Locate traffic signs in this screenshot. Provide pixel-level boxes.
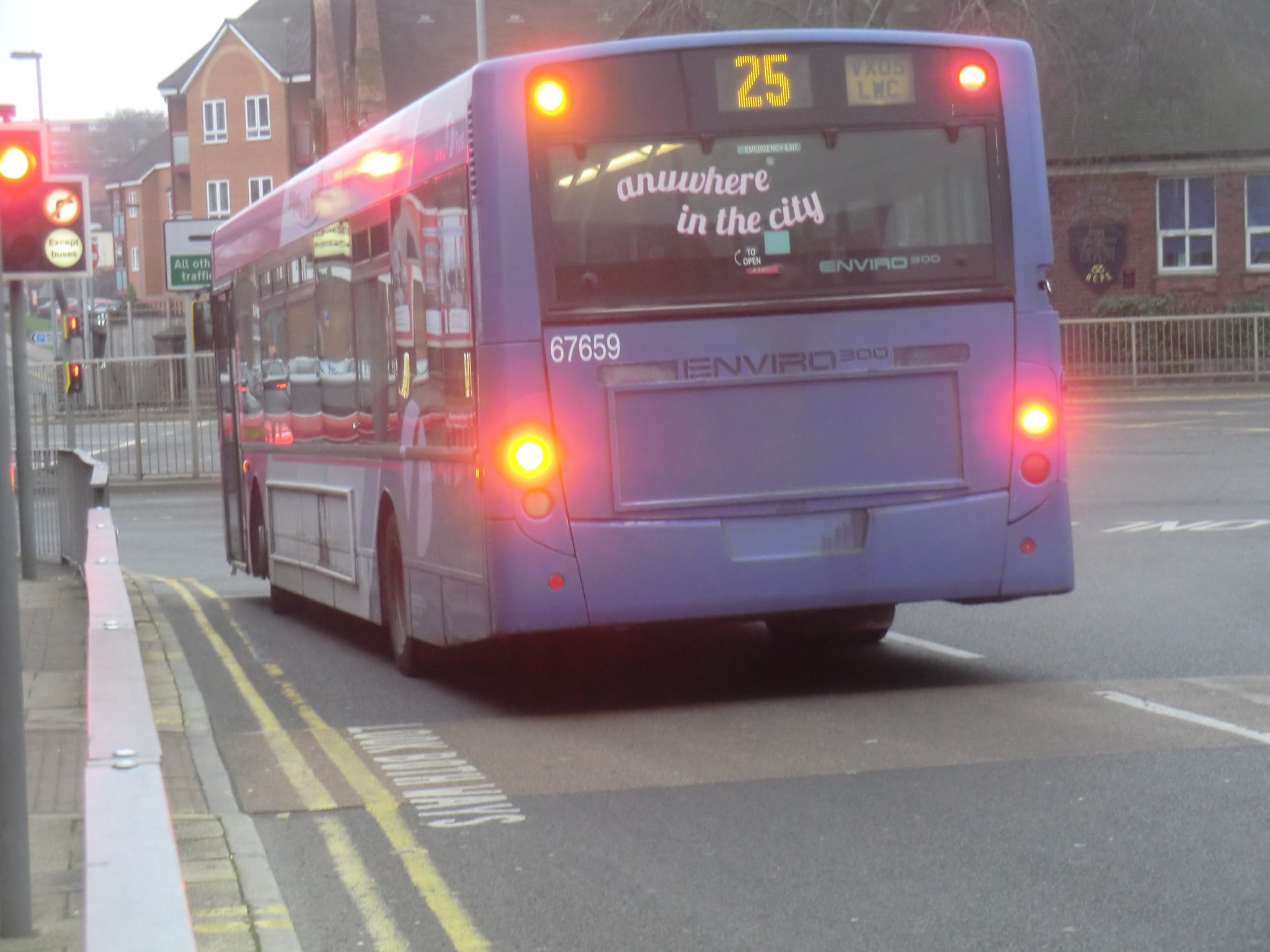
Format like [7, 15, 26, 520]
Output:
[163, 217, 229, 291]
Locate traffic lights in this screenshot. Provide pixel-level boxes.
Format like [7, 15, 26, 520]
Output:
[0, 119, 50, 281]
[70, 364, 83, 391]
[69, 316, 81, 337]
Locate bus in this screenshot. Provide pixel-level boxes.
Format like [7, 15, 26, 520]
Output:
[189, 26, 1075, 676]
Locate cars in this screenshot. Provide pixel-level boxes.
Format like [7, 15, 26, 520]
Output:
[36, 296, 157, 325]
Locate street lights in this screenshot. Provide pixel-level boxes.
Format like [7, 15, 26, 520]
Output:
[11, 51, 45, 119]
